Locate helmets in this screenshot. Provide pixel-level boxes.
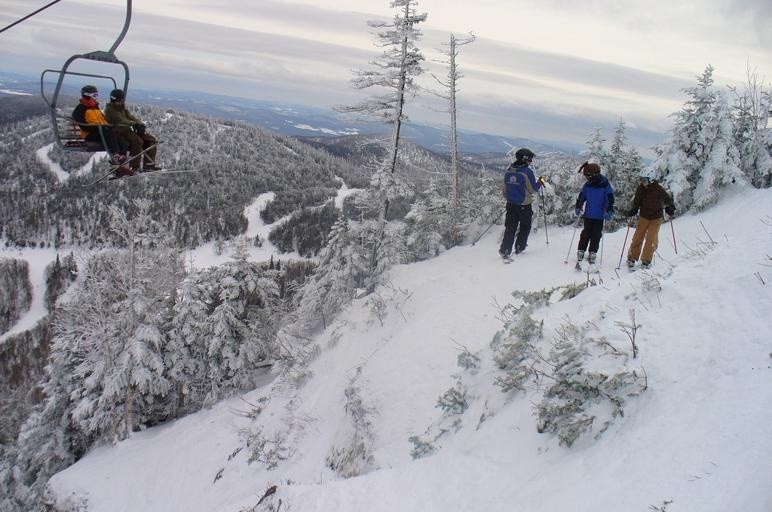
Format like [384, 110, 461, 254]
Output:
[583, 164, 600, 177]
[516, 149, 534, 161]
[640, 167, 654, 183]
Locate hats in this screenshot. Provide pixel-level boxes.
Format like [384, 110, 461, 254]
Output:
[111, 89, 125, 100]
[81, 86, 97, 98]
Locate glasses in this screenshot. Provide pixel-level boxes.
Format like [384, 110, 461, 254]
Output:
[85, 93, 98, 99]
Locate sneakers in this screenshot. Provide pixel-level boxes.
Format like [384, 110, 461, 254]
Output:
[578, 251, 596, 259]
[144, 165, 161, 171]
[627, 260, 649, 267]
[501, 248, 522, 258]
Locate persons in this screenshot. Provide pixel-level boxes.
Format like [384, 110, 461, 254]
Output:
[497, 147, 547, 258]
[71, 84, 134, 177]
[102, 88, 163, 173]
[574, 163, 616, 264]
[626, 165, 678, 268]
